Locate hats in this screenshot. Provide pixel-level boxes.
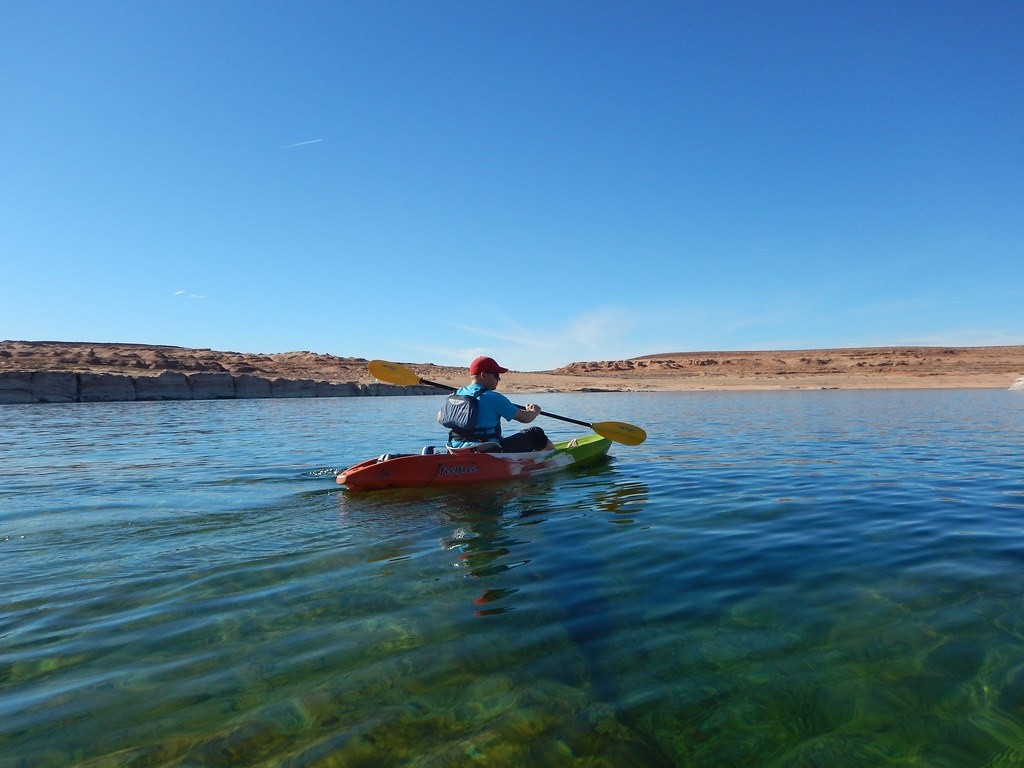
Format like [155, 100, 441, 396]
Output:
[470, 356, 508, 375]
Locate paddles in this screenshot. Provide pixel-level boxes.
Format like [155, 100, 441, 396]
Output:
[368, 360, 647, 446]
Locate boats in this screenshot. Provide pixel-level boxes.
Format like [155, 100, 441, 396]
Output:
[336, 434, 612, 493]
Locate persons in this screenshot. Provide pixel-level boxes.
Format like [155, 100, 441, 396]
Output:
[447, 356, 578, 453]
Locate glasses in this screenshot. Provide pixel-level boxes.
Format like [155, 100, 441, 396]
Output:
[485, 372, 499, 379]
